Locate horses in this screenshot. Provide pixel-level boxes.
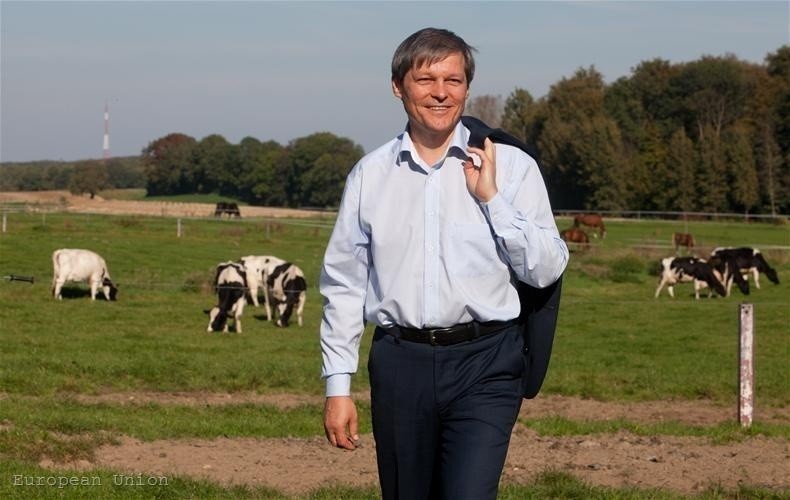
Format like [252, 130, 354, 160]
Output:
[214, 202, 241, 219]
[671, 232, 697, 255]
[560, 213, 607, 254]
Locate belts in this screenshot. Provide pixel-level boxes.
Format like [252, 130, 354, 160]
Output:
[395, 324, 479, 345]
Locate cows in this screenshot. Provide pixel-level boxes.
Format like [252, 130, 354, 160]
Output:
[50, 248, 118, 301]
[202, 254, 308, 335]
[653, 247, 779, 300]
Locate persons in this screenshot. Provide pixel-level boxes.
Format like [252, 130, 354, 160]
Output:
[321, 27, 568, 500]
